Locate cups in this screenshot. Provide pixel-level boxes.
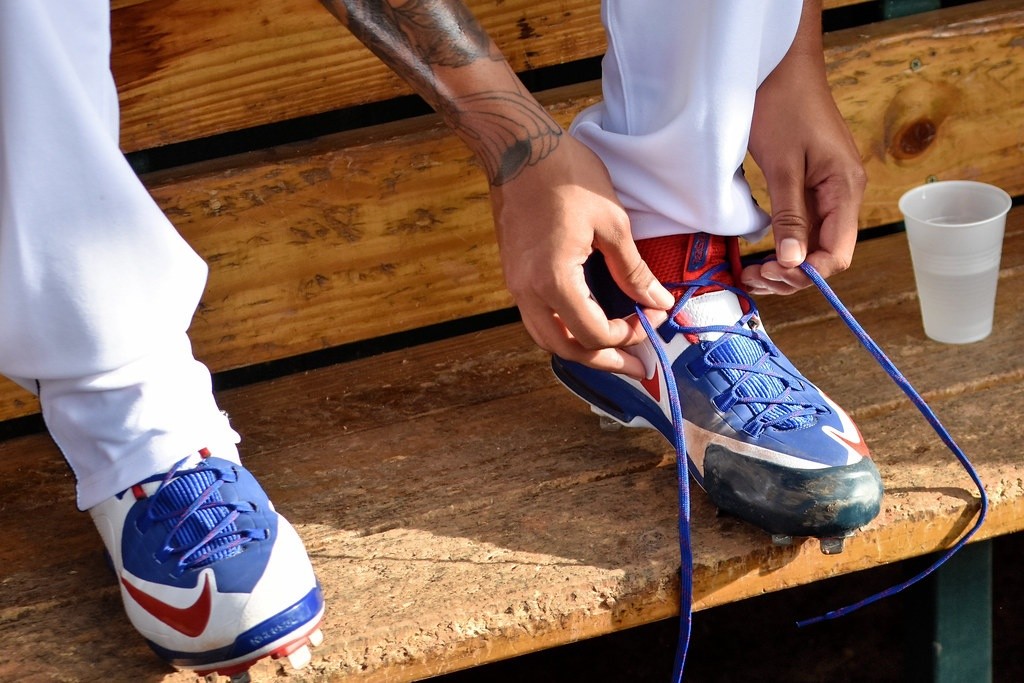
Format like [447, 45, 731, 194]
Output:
[898, 179, 1013, 343]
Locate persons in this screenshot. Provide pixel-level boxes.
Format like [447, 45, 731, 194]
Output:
[0, 0, 885, 678]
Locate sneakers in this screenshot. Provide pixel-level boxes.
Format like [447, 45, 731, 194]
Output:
[546, 229, 884, 553]
[86, 439, 326, 680]
